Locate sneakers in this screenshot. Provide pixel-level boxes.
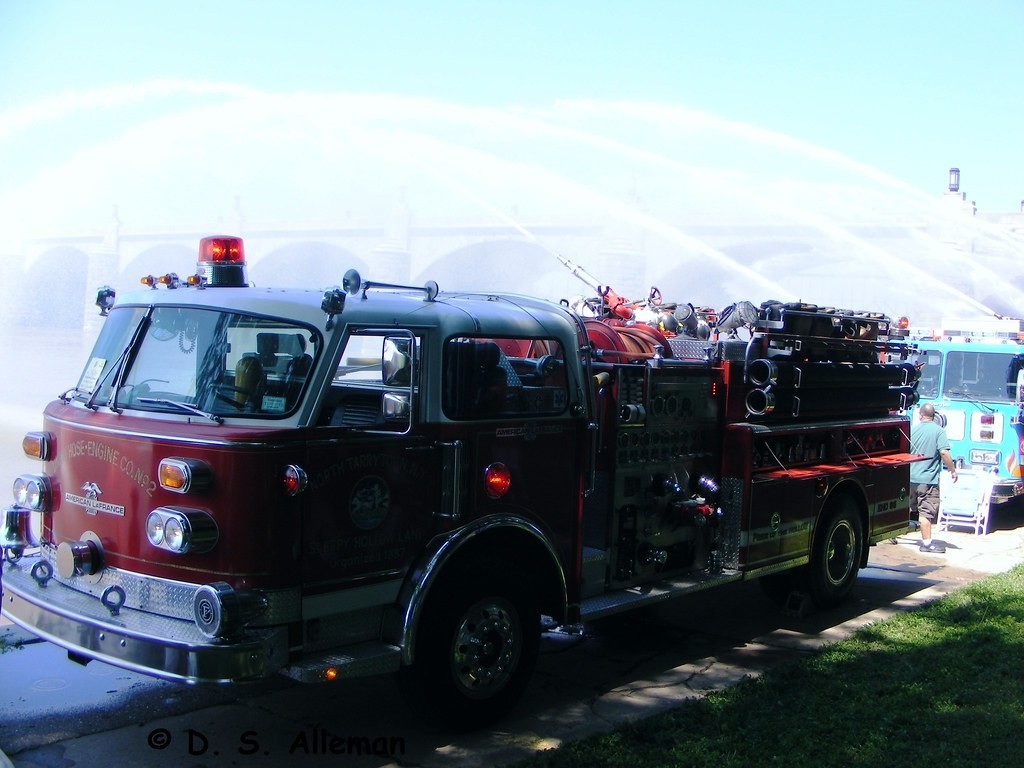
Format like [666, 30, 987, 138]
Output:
[889, 534, 898, 544]
[920, 540, 946, 553]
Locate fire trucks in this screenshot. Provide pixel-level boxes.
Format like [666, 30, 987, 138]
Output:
[876, 311, 1024, 537]
[0, 232, 934, 738]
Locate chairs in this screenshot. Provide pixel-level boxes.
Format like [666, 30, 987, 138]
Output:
[939, 468, 994, 537]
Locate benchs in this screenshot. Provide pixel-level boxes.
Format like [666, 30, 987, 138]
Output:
[205, 374, 411, 435]
